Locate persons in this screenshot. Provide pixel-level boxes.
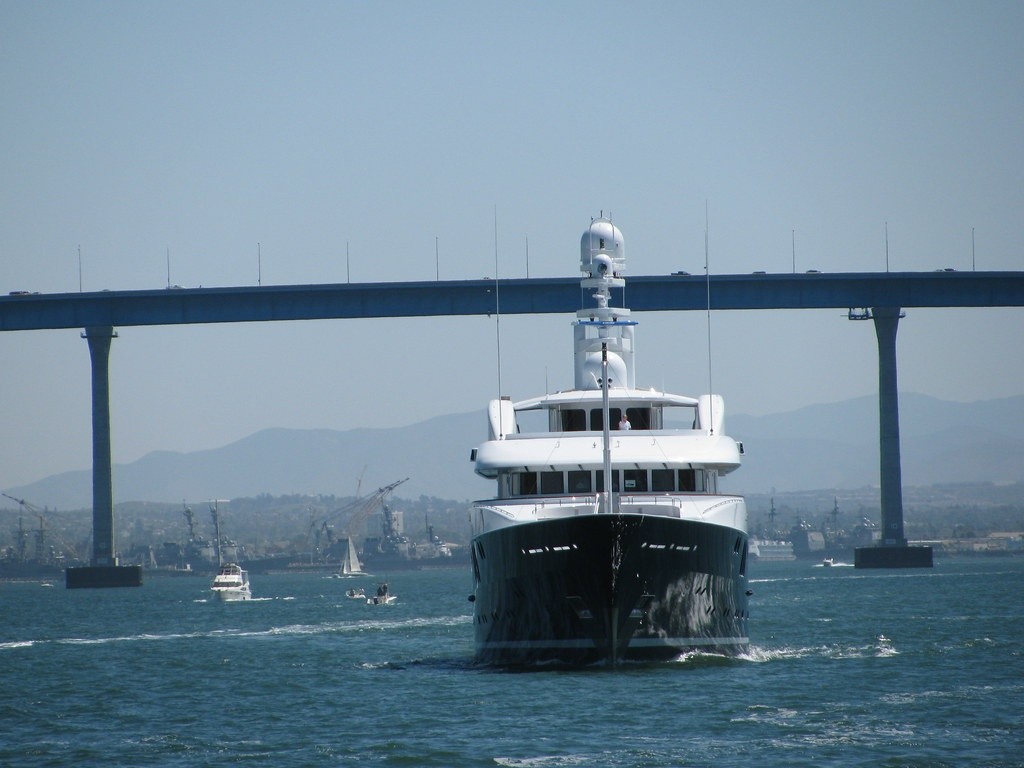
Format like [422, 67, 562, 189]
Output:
[618, 414, 632, 430]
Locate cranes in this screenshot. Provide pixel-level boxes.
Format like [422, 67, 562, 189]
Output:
[0, 494, 85, 557]
[305, 474, 416, 552]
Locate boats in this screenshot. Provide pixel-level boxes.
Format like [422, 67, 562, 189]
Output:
[823, 558, 833, 567]
[364, 580, 397, 605]
[345, 589, 365, 600]
[207, 563, 253, 604]
[464, 204, 748, 667]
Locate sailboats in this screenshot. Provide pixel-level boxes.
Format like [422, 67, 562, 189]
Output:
[332, 539, 368, 578]
